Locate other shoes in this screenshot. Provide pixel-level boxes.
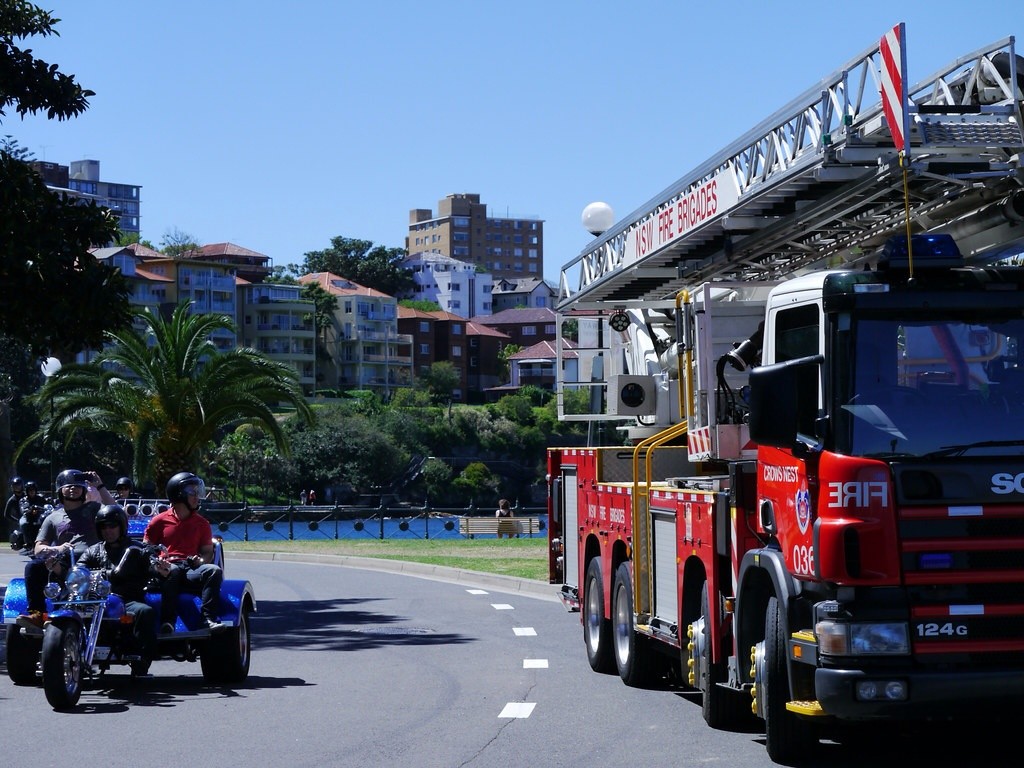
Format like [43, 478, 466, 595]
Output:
[206, 619, 227, 634]
[160, 623, 174, 637]
[19, 547, 34, 556]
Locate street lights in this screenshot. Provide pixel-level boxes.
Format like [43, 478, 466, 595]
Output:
[581, 201, 614, 446]
[41, 357, 62, 504]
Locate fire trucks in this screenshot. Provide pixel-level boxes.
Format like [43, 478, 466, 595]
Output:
[543, 22, 1024, 764]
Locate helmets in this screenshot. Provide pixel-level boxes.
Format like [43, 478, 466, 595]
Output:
[166, 471, 206, 503]
[10, 475, 25, 486]
[25, 481, 37, 493]
[115, 476, 134, 490]
[95, 504, 128, 541]
[55, 469, 88, 493]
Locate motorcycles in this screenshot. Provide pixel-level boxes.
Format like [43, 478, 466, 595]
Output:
[1, 538, 263, 709]
[117, 498, 173, 542]
[9, 504, 61, 559]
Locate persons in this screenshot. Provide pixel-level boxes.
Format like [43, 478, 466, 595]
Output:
[143, 471, 227, 634]
[114, 477, 143, 499]
[19, 481, 47, 550]
[16, 469, 129, 634]
[4, 476, 27, 524]
[310, 490, 316, 505]
[300, 490, 307, 506]
[496, 499, 514, 538]
[45, 505, 171, 681]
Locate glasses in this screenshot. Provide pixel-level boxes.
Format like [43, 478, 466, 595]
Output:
[117, 486, 129, 491]
[185, 490, 200, 496]
[100, 521, 119, 530]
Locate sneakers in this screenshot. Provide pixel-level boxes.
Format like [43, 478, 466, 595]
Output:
[16, 610, 45, 634]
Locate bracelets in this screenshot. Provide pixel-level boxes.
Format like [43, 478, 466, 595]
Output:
[97, 485, 104, 490]
